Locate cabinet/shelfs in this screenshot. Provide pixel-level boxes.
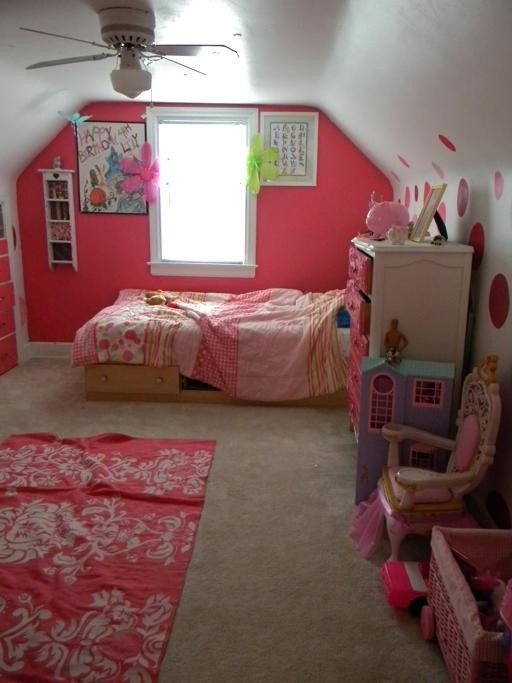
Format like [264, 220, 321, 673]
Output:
[39, 167, 78, 270]
[0, 239, 18, 375]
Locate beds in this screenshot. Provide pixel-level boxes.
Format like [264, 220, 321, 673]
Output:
[67, 287, 350, 408]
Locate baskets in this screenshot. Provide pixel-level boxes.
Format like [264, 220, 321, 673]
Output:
[428, 526, 512, 683]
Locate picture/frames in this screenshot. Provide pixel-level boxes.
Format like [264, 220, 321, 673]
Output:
[77, 119, 149, 215]
[408, 181, 449, 244]
[256, 110, 317, 186]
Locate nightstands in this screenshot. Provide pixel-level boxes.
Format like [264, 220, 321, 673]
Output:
[343, 236, 476, 443]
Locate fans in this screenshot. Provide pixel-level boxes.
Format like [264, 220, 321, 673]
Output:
[18, 1, 243, 100]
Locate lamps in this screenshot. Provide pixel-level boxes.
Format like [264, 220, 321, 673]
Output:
[111, 48, 151, 100]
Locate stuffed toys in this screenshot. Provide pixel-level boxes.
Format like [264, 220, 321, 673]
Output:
[144, 290, 181, 305]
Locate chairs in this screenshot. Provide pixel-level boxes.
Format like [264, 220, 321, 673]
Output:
[348, 354, 502, 562]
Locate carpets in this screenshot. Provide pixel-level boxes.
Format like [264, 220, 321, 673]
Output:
[1, 431, 216, 682]
